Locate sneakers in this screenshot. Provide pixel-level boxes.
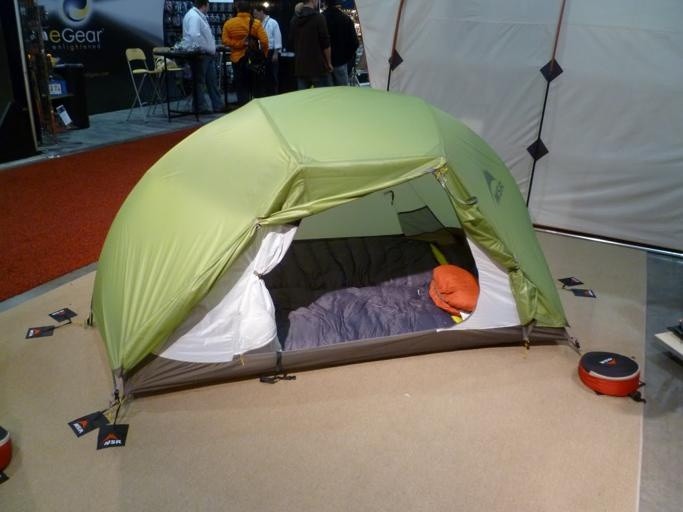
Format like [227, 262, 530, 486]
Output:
[192, 105, 235, 114]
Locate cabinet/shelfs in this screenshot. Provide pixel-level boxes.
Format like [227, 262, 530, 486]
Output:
[46, 63, 90, 133]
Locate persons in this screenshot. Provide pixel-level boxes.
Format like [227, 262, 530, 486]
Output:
[180, 1, 223, 115]
[219, 1, 370, 113]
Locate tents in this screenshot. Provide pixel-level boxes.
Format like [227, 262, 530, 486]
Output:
[86, 85, 587, 406]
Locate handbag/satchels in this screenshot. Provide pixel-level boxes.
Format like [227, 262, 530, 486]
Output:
[248, 49, 266, 69]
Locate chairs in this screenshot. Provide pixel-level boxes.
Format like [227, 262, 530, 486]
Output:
[125, 44, 226, 124]
